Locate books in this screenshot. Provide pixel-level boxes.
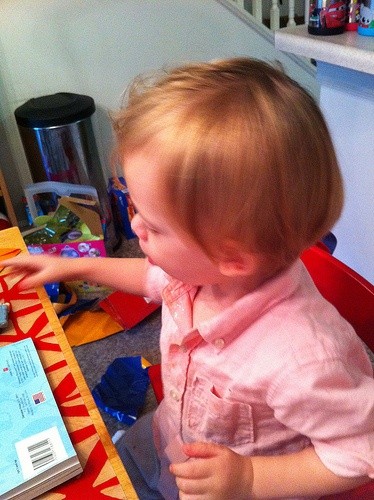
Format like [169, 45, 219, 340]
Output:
[1, 335, 85, 500]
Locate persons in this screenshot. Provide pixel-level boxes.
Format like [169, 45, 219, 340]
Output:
[0, 53, 374, 499]
